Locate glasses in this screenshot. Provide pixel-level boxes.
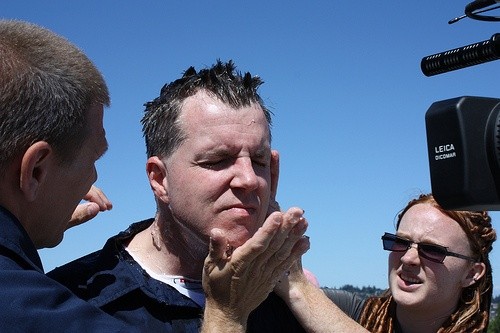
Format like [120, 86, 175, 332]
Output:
[381, 232, 478, 263]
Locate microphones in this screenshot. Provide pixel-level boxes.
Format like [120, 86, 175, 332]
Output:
[421, 33, 500, 77]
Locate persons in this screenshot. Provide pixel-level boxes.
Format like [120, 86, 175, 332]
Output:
[0, 15, 312, 333]
[42, 57, 305, 333]
[265, 150, 498, 333]
